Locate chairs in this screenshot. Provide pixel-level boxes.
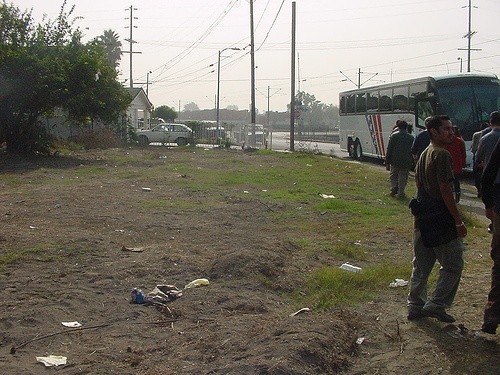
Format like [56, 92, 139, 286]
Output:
[351, 95, 407, 110]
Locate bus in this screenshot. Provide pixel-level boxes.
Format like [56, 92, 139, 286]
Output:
[338, 71, 500, 172]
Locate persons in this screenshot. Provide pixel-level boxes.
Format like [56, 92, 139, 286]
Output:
[470, 111, 500, 335]
[406, 115, 467, 323]
[386, 120, 414, 198]
[410, 117, 432, 163]
[445, 136, 466, 202]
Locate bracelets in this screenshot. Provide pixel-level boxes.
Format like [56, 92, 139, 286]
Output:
[456, 221, 464, 226]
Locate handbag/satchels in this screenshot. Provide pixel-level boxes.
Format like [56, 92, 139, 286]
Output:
[412, 198, 457, 247]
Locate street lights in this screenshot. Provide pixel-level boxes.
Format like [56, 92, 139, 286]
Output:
[217, 47, 240, 145]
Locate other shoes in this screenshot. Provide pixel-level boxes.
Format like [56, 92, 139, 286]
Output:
[399, 194, 404, 198]
[390, 192, 397, 196]
[420, 308, 455, 322]
[481, 321, 498, 334]
[407, 312, 420, 320]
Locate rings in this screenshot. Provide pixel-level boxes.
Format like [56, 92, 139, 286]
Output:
[464, 233, 466, 236]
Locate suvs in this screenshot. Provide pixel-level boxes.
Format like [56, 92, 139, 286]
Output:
[135, 123, 192, 147]
[200, 121, 225, 139]
[240, 124, 266, 145]
